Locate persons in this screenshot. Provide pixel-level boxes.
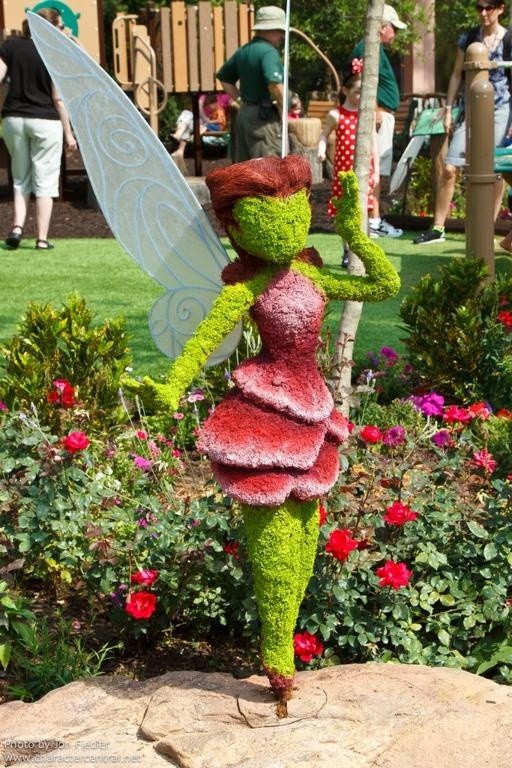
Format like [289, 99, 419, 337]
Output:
[168, 90, 303, 158]
[341, 4, 408, 239]
[316, 61, 376, 269]
[215, 5, 289, 163]
[0, 8, 79, 250]
[412, 0, 512, 246]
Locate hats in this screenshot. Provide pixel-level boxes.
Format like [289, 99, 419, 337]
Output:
[251, 6, 293, 32]
[383, 3, 408, 29]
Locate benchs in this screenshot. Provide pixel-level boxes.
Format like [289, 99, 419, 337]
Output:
[303, 99, 414, 159]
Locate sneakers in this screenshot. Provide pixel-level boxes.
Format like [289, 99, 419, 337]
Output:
[369, 218, 403, 239]
[413, 226, 446, 244]
[342, 250, 349, 267]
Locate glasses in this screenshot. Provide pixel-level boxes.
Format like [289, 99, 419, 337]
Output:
[476, 5, 496, 11]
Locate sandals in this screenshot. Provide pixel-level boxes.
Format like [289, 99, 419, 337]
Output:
[5, 226, 24, 246]
[35, 239, 54, 249]
